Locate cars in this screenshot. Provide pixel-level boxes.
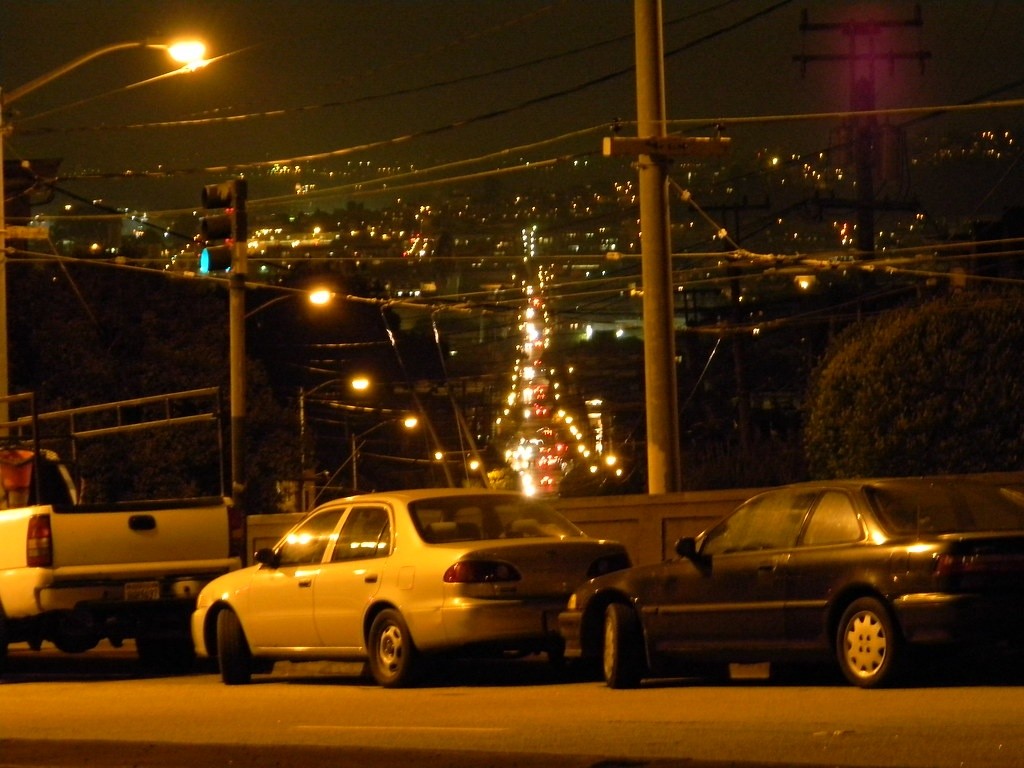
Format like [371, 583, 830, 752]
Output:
[190, 488, 634, 689]
[554, 476, 1024, 692]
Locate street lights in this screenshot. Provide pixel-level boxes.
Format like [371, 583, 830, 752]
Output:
[0, 36, 207, 440]
[294, 373, 370, 514]
[348, 411, 421, 501]
[229, 177, 339, 508]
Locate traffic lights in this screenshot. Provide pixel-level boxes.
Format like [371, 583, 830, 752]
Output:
[199, 182, 233, 277]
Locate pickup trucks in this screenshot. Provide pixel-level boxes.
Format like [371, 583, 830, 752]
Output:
[0, 385, 250, 676]
